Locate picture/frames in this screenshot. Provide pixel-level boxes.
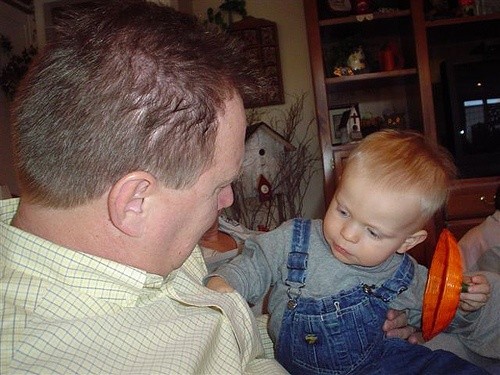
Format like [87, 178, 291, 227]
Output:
[328, 107, 349, 145]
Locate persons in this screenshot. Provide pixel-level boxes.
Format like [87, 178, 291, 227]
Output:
[1, 0, 420, 375]
[201, 126, 491, 375]
[458, 183, 500, 275]
[197, 210, 273, 318]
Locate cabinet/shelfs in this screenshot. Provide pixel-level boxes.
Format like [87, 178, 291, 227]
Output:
[303, 0, 500, 268]
